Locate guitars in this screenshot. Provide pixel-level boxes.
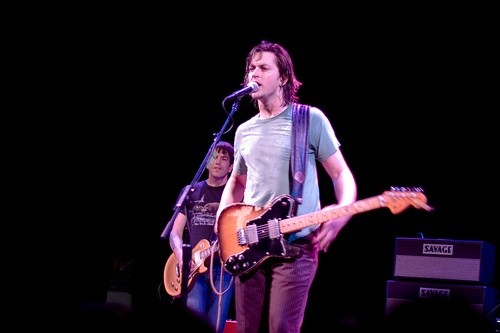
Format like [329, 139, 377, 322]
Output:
[215, 185, 433, 277]
[162, 235, 219, 296]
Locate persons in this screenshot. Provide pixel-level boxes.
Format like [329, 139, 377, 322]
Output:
[213, 42, 356, 333]
[167, 142, 242, 331]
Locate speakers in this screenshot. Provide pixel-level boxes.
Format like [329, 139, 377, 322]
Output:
[391, 235, 494, 284]
[384, 281, 496, 317]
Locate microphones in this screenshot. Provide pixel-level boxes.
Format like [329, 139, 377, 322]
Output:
[225, 81, 258, 101]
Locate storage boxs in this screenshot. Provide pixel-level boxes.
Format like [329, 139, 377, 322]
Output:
[392, 235, 497, 284]
[385, 277, 498, 326]
